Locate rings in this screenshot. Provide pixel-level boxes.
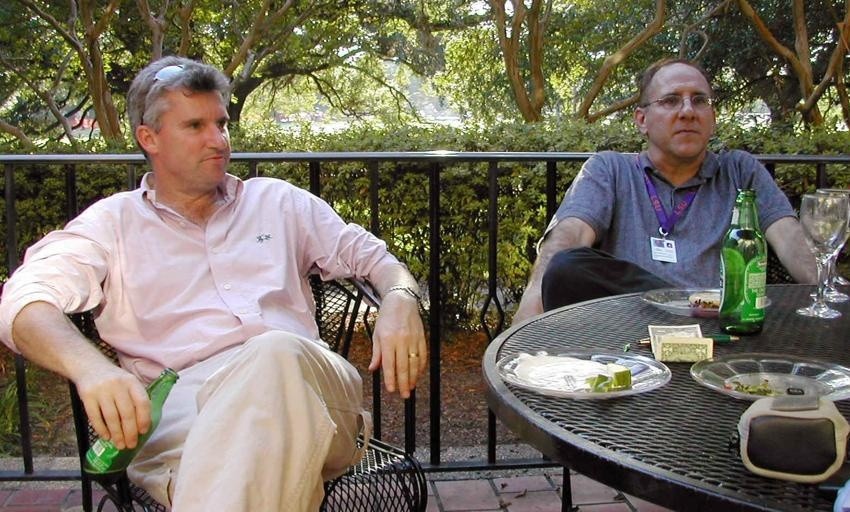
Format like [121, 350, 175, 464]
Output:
[406, 351, 419, 359]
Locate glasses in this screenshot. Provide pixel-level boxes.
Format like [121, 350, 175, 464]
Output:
[151, 64, 184, 82]
[644, 94, 716, 112]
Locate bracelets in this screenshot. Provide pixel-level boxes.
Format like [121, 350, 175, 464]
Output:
[387, 282, 423, 302]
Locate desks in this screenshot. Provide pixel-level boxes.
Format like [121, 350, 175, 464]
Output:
[481, 283, 850, 512]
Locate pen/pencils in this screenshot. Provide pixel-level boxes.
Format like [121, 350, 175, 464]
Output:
[637, 334, 740, 347]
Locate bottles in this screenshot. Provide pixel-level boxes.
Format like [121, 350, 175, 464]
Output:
[719, 188, 768, 336]
[80, 366, 179, 488]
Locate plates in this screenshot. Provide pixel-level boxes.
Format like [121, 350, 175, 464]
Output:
[692, 352, 850, 404]
[643, 288, 774, 318]
[495, 349, 673, 403]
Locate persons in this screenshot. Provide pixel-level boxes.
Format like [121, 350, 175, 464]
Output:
[510, 57, 836, 327]
[0, 56, 429, 512]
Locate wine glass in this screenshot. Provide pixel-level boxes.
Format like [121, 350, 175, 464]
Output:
[794, 188, 850, 321]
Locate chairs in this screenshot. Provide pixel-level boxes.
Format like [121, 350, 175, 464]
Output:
[68, 270, 429, 512]
